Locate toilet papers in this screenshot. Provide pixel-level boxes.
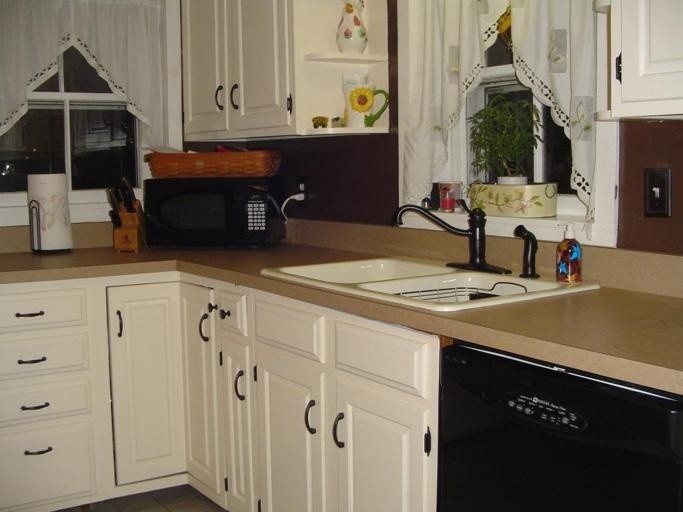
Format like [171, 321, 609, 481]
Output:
[27, 173, 74, 251]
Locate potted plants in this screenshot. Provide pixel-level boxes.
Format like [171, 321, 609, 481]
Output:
[469, 92, 559, 185]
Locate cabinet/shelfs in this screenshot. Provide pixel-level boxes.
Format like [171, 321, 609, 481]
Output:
[179, 2, 389, 144]
[593, 0, 683, 121]
[254, 356, 427, 511]
[215, 287, 253, 512]
[1, 290, 99, 511]
[104, 281, 183, 494]
[184, 282, 215, 487]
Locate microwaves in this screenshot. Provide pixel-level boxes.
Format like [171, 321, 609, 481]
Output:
[141, 177, 286, 250]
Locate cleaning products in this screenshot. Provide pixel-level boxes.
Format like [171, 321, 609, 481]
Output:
[555, 222, 584, 285]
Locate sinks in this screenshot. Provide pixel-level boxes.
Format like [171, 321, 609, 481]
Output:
[351, 270, 602, 311]
[258, 253, 460, 284]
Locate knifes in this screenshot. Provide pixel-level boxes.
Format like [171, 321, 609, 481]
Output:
[106, 176, 136, 228]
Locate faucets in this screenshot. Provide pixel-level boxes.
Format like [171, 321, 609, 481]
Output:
[393, 196, 485, 264]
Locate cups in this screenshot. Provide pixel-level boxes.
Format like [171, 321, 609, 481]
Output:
[341, 83, 390, 128]
[430, 181, 463, 213]
[312, 116, 329, 128]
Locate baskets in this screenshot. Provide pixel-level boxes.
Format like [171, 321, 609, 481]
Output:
[142, 147, 286, 179]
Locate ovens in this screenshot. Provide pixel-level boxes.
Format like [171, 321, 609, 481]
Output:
[438, 344, 682, 512]
